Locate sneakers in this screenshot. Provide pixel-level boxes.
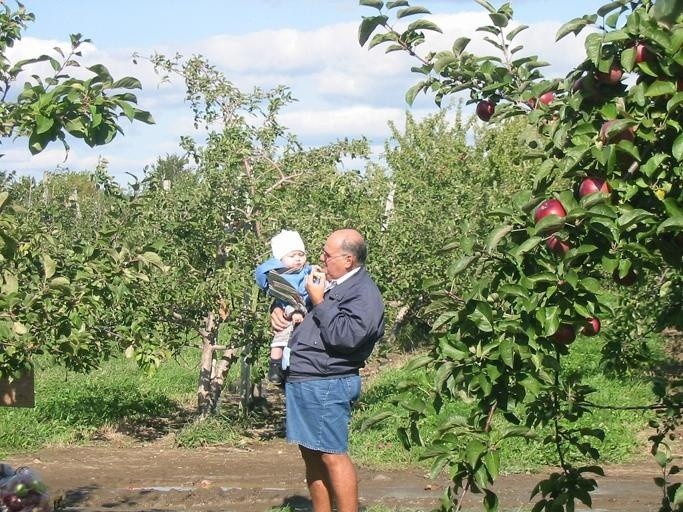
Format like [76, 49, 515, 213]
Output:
[268, 362, 289, 386]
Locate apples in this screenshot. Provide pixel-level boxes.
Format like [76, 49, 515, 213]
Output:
[580, 316, 601, 336]
[539, 91, 557, 106]
[549, 324, 576, 343]
[629, 44, 647, 63]
[535, 198, 567, 224]
[545, 233, 572, 254]
[579, 177, 611, 198]
[573, 76, 603, 103]
[477, 101, 495, 122]
[6, 480, 47, 511]
[612, 267, 639, 287]
[600, 119, 636, 145]
[596, 65, 623, 85]
[528, 97, 538, 109]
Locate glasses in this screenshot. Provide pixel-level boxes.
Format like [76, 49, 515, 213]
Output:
[322, 249, 346, 260]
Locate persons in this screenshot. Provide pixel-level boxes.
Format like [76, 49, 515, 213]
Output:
[256, 229, 385, 512]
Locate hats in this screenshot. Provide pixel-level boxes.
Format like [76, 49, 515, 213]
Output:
[270, 229, 306, 260]
